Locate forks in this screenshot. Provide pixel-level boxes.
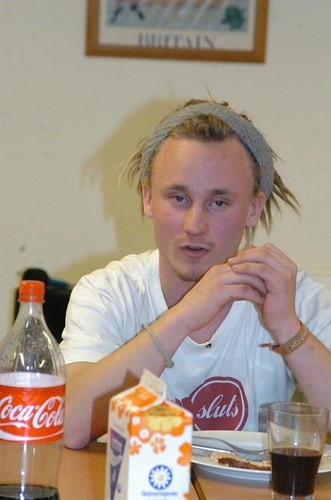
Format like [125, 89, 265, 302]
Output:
[192, 444, 268, 462]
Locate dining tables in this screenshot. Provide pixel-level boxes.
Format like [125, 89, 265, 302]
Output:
[1, 435, 331, 499]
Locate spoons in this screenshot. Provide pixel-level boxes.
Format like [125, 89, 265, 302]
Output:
[191, 436, 268, 454]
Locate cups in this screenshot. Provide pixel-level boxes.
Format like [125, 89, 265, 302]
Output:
[267, 403, 327, 500]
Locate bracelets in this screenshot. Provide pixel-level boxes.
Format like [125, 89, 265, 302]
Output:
[143, 324, 174, 368]
[258, 320, 310, 356]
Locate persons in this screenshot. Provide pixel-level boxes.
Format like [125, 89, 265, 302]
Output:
[53, 98, 331, 500]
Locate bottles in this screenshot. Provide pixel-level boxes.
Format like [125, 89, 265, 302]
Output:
[0, 282, 68, 499]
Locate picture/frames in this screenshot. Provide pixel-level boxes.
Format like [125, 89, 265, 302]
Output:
[85, 0, 268, 62]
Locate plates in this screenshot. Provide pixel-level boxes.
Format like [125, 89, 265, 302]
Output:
[190, 430, 331, 480]
